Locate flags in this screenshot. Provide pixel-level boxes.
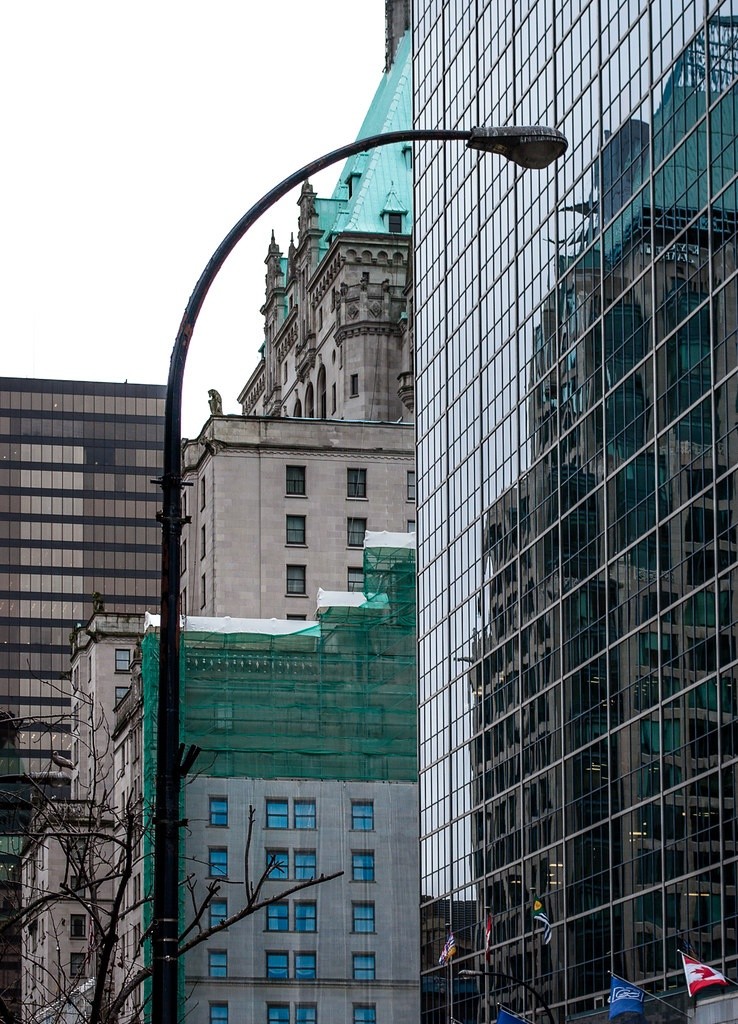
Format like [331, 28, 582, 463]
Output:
[484, 909, 492, 961]
[609, 974, 645, 1022]
[439, 932, 457, 967]
[532, 892, 553, 944]
[496, 1009, 529, 1024]
[682, 954, 729, 997]
[682, 938, 705, 963]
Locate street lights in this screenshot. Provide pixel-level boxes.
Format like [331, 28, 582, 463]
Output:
[153, 126, 568, 1024]
[457, 969, 554, 1024]
